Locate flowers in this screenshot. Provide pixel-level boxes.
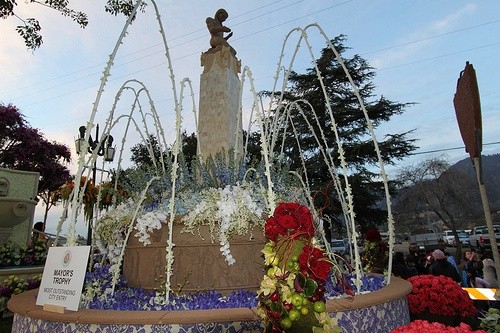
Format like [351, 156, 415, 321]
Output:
[390, 320, 485, 333]
[89, 152, 304, 283]
[83, 264, 260, 310]
[404, 275, 477, 326]
[0, 275, 43, 322]
[0, 240, 53, 268]
[359, 228, 390, 275]
[254, 203, 354, 333]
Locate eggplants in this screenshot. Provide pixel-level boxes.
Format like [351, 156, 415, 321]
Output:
[276, 272, 290, 279]
[295, 277, 304, 292]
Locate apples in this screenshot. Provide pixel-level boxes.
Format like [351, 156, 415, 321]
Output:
[266, 254, 301, 277]
[269, 278, 326, 328]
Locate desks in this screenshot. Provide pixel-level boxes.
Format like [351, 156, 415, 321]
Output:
[462, 288, 500, 313]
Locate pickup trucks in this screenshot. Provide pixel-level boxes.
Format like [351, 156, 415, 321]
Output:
[469, 225, 500, 254]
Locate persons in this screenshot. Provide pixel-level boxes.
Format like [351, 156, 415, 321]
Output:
[206, 8, 236, 56]
[332, 245, 500, 312]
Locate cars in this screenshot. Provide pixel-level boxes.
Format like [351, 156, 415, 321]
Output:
[442, 230, 461, 246]
[329, 232, 396, 257]
[409, 233, 444, 255]
[455, 232, 471, 248]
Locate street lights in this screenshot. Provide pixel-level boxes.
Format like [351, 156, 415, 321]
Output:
[74, 123, 115, 246]
